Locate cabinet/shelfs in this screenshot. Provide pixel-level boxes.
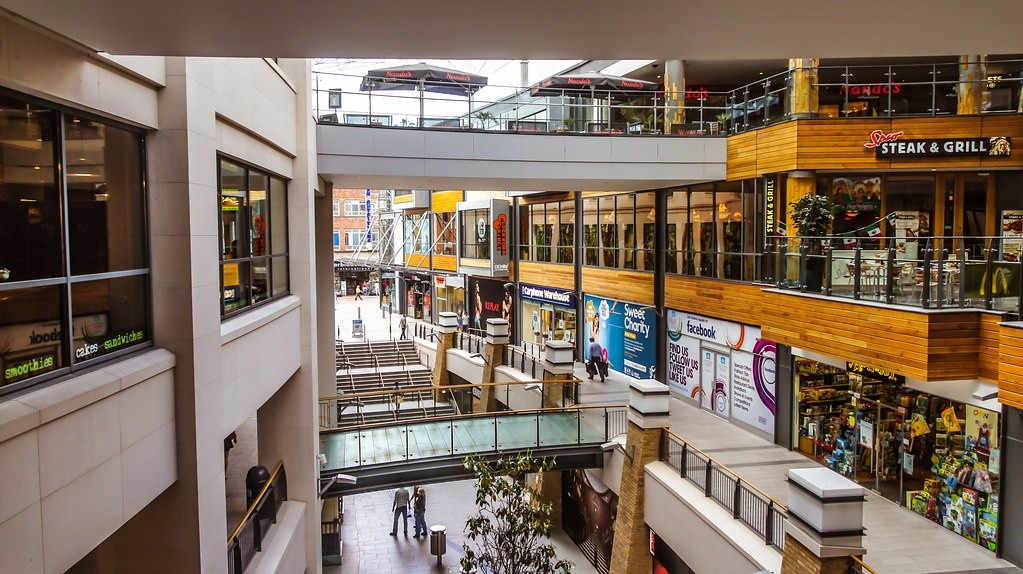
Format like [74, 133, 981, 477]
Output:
[798, 372, 922, 458]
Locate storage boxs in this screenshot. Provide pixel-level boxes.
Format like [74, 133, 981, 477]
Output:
[801, 363, 999, 553]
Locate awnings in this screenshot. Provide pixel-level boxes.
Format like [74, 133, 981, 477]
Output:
[334, 257, 379, 272]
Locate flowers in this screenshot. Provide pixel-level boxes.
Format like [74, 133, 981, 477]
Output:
[789, 191, 843, 255]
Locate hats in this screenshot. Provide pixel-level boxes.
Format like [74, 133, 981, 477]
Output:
[417, 488, 425, 495]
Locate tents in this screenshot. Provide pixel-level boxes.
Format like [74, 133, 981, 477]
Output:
[530, 69, 659, 121]
[360, 61, 488, 125]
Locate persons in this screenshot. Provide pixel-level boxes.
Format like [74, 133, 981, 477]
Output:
[477, 238, 487, 257]
[355, 285, 364, 301]
[456, 312, 463, 330]
[366, 280, 371, 296]
[590, 313, 600, 343]
[588, 337, 605, 382]
[462, 311, 469, 334]
[474, 281, 482, 337]
[223, 432, 238, 478]
[502, 288, 512, 340]
[410, 485, 427, 539]
[398, 314, 406, 340]
[390, 381, 404, 412]
[390, 488, 411, 537]
[441, 240, 453, 255]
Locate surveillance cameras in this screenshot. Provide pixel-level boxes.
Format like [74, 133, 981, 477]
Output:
[652, 65, 658, 68]
[319, 454, 327, 465]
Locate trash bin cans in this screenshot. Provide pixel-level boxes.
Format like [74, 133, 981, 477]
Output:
[245, 465, 276, 521]
[429, 525, 447, 556]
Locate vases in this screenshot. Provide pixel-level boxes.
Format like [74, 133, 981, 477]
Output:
[800, 256, 826, 292]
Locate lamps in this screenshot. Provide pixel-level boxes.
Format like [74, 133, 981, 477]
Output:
[316, 474, 358, 499]
[66, 122, 103, 177]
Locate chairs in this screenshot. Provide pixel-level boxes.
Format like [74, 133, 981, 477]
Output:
[846, 258, 961, 305]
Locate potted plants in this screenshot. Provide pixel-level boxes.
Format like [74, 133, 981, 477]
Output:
[716, 112, 732, 135]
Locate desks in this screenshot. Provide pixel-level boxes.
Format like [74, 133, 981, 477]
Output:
[862, 265, 904, 294]
[922, 268, 960, 304]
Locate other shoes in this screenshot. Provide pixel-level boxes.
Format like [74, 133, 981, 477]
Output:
[421, 532, 427, 535]
[390, 532, 397, 536]
[404, 531, 407, 535]
[601, 378, 604, 382]
[413, 535, 420, 538]
[413, 526, 416, 528]
[588, 377, 593, 380]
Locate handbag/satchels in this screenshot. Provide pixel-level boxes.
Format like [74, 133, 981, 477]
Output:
[601, 362, 609, 377]
[585, 362, 598, 375]
